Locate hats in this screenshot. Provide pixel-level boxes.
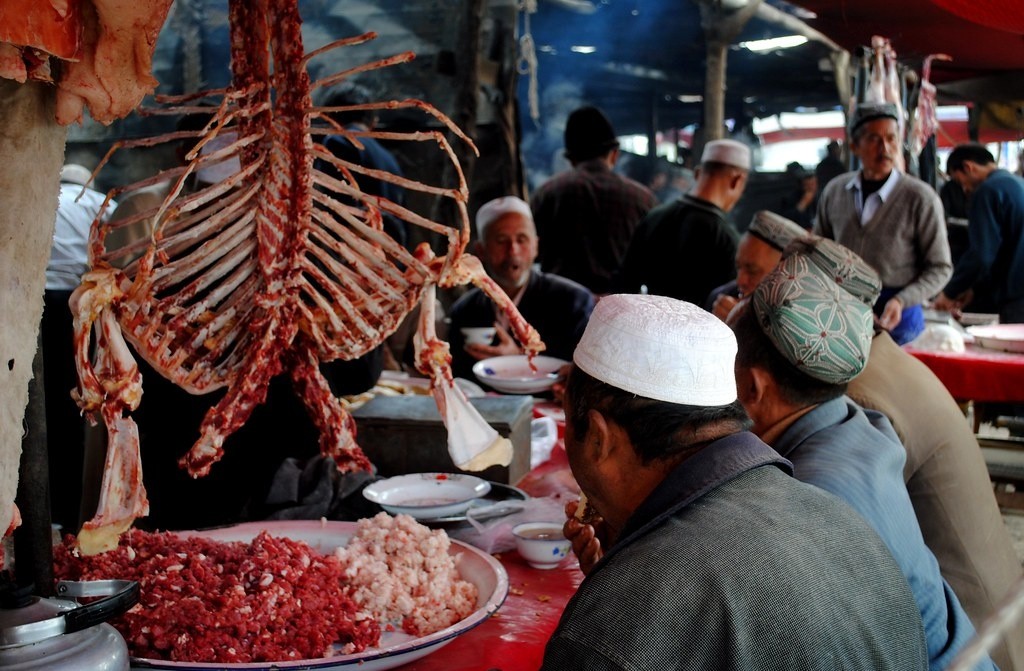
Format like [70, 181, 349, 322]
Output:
[848, 102, 899, 135]
[753, 251, 873, 384]
[748, 209, 810, 251]
[780, 233, 883, 306]
[475, 196, 533, 242]
[701, 138, 751, 171]
[565, 106, 619, 161]
[573, 294, 740, 407]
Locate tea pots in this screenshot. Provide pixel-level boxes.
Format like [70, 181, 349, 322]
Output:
[0, 567, 141, 670]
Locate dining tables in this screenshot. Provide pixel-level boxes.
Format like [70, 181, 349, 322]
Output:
[129, 462, 587, 671]
[902, 320, 1024, 497]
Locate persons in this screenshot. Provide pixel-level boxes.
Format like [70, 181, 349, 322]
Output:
[632, 141, 754, 312]
[721, 255, 999, 671]
[936, 138, 1024, 322]
[441, 192, 601, 391]
[712, 210, 812, 325]
[535, 293, 931, 671]
[530, 106, 659, 299]
[132, 134, 400, 533]
[811, 96, 954, 349]
[621, 117, 1024, 242]
[751, 234, 1024, 671]
[106, 103, 267, 300]
[36, 165, 120, 534]
[316, 84, 400, 236]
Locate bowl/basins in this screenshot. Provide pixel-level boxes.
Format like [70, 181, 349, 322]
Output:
[461, 327, 499, 348]
[512, 522, 572, 571]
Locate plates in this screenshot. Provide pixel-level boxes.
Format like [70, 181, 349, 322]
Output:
[409, 479, 532, 530]
[360, 469, 493, 520]
[965, 321, 1024, 354]
[475, 353, 573, 395]
[122, 513, 510, 670]
